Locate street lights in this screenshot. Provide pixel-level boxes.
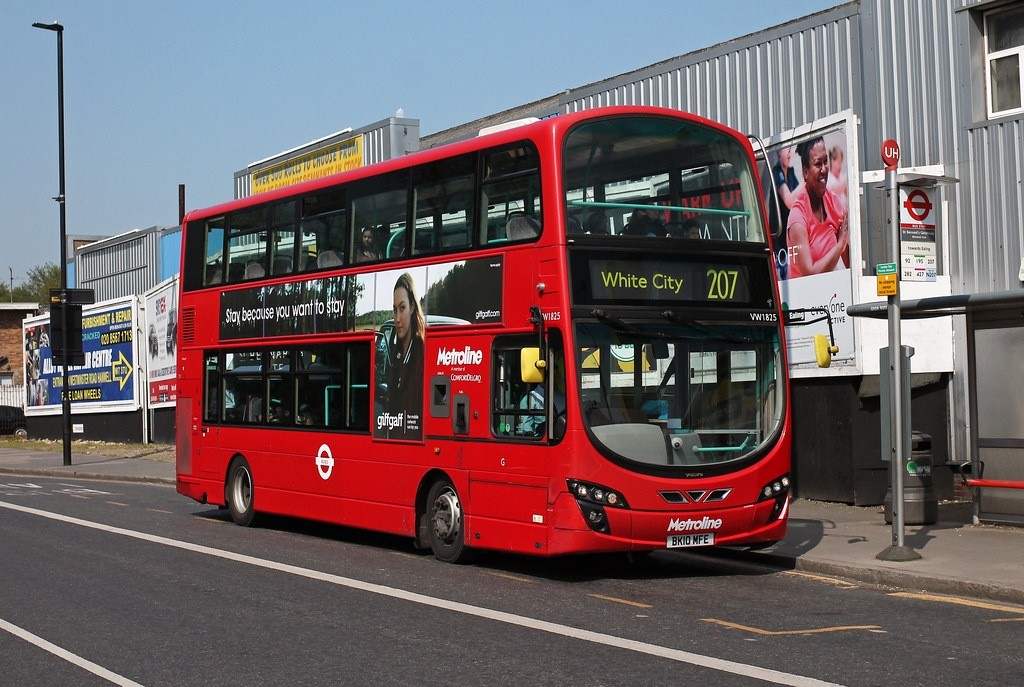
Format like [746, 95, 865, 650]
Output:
[32, 21, 72, 465]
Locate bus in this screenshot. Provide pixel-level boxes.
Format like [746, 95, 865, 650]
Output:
[177, 105, 840, 564]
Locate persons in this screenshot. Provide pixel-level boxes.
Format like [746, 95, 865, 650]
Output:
[624, 201, 669, 237]
[380, 273, 424, 441]
[213, 369, 245, 411]
[355, 228, 382, 262]
[587, 213, 607, 233]
[682, 220, 701, 238]
[519, 364, 566, 436]
[768, 137, 851, 282]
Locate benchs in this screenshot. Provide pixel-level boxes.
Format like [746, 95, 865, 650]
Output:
[958, 460, 1024, 490]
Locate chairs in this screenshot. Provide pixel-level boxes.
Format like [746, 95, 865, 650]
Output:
[245, 391, 368, 427]
[208, 220, 686, 288]
[507, 382, 532, 434]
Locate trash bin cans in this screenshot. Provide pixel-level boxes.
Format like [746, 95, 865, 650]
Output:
[884, 431, 938, 525]
